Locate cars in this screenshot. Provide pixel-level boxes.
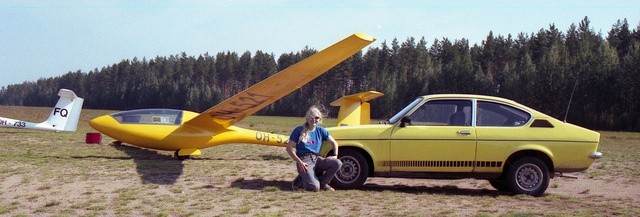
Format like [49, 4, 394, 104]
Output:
[318, 93, 602, 196]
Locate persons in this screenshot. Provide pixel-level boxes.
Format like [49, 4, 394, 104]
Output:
[286, 106, 343, 193]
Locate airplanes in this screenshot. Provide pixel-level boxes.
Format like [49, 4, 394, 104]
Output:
[0, 89, 85, 132]
[88, 33, 384, 159]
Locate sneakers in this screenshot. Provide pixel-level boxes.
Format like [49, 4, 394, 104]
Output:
[292, 179, 303, 192]
[320, 184, 334, 191]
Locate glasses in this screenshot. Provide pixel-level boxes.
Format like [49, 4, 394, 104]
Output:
[315, 116, 319, 120]
